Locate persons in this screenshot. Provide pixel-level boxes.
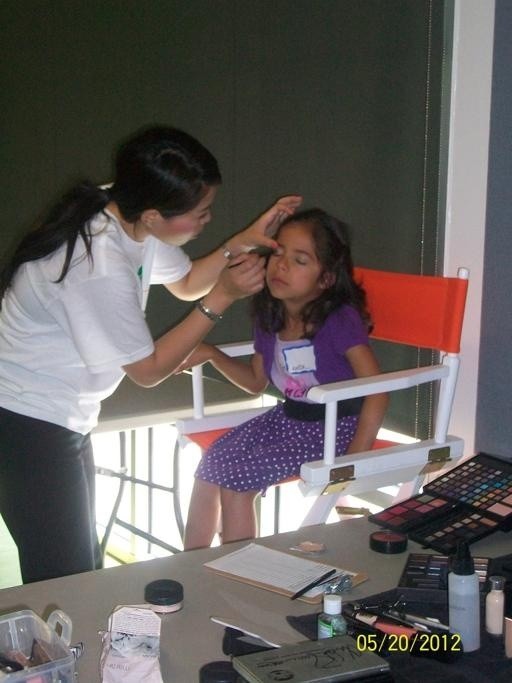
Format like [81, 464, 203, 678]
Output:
[0, 126, 303, 584]
[173, 207, 391, 551]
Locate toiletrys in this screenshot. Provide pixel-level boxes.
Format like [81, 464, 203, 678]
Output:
[447, 540, 481, 654]
[144, 579, 184, 614]
[485, 575, 506, 635]
[370, 532, 408, 554]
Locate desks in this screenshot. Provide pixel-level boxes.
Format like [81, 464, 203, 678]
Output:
[1, 514, 511, 683]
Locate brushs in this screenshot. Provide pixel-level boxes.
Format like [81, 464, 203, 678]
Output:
[382, 608, 450, 633]
[228, 250, 277, 269]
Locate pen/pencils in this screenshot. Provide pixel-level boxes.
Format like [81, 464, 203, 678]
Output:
[290, 569, 336, 600]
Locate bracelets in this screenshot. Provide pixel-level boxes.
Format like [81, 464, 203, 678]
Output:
[220, 244, 233, 260]
[196, 300, 224, 322]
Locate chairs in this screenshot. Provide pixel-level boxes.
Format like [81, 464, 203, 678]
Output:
[172, 267, 472, 529]
